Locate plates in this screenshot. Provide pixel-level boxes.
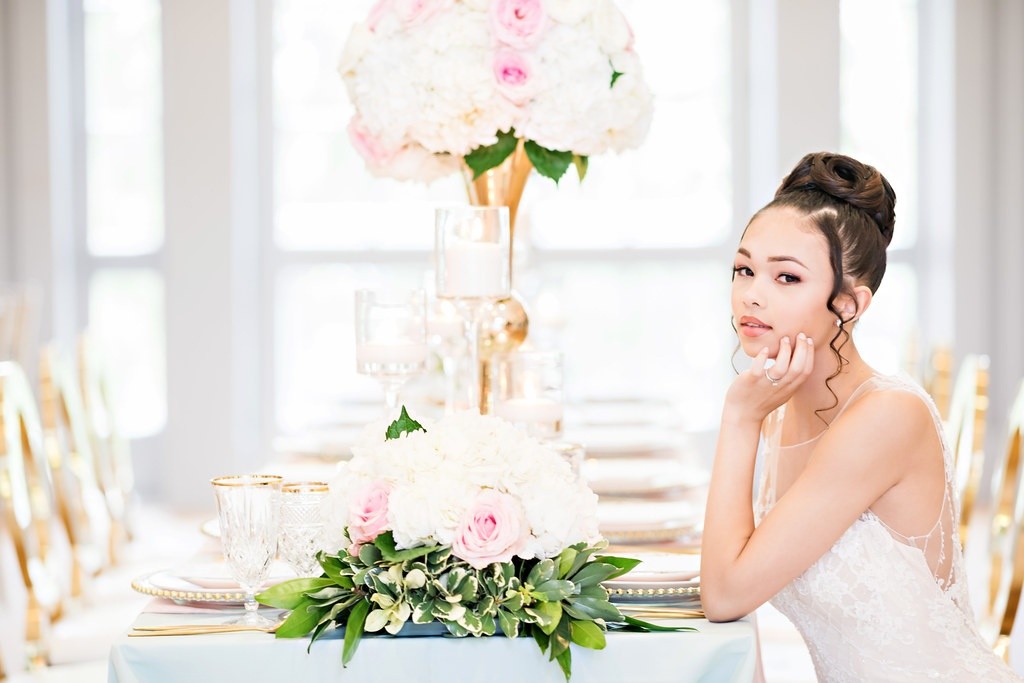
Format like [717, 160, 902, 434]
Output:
[201, 516, 221, 539]
[587, 551, 701, 603]
[131, 571, 269, 607]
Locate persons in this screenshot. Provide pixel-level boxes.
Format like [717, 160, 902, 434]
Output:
[699, 152, 1024, 683]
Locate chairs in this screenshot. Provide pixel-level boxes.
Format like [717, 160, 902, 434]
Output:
[902, 350, 1024, 665]
[0, 337, 195, 683]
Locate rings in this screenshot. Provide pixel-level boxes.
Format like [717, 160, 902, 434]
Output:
[765, 369, 779, 386]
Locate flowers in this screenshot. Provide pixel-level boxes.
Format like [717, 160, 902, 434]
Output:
[257, 395, 701, 683]
[340, 0, 653, 187]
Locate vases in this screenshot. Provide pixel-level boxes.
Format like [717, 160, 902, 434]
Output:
[463, 135, 533, 415]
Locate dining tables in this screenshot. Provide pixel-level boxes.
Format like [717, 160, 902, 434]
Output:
[108, 424, 760, 683]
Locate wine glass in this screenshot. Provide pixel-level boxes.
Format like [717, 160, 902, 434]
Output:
[278, 481, 331, 578]
[211, 474, 284, 629]
